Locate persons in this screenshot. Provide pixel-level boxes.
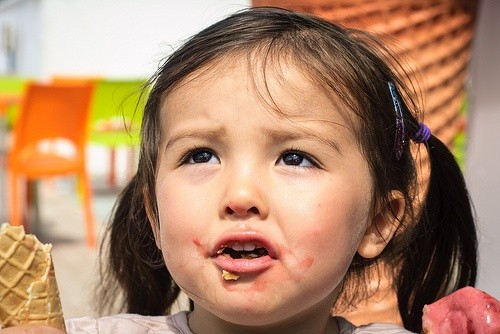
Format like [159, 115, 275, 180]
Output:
[0, 5, 482, 334]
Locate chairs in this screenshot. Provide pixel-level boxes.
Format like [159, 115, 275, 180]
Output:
[1, 77, 152, 252]
